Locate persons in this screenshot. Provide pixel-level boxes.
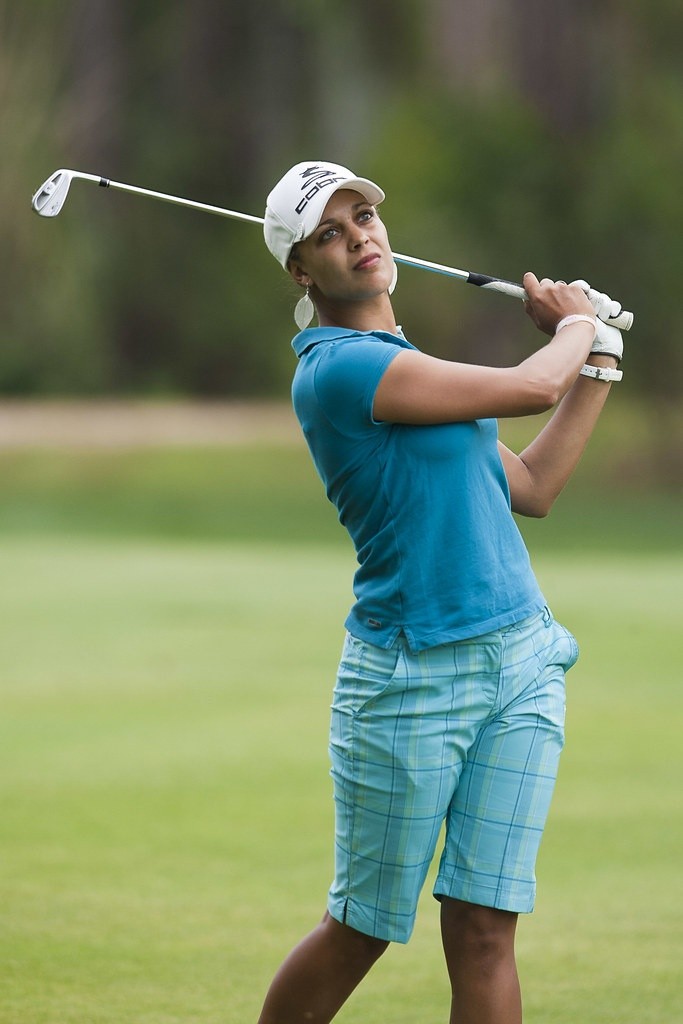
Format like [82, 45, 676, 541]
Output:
[257, 160, 625, 1024]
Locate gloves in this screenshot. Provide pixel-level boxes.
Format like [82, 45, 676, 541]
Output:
[568, 280, 623, 364]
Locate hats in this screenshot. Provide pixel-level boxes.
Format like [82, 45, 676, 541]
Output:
[263, 161, 386, 273]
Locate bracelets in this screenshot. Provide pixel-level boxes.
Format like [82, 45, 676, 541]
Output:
[555, 314, 597, 342]
[580, 365, 623, 382]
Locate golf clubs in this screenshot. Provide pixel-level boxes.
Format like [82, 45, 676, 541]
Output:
[30, 168, 636, 333]
